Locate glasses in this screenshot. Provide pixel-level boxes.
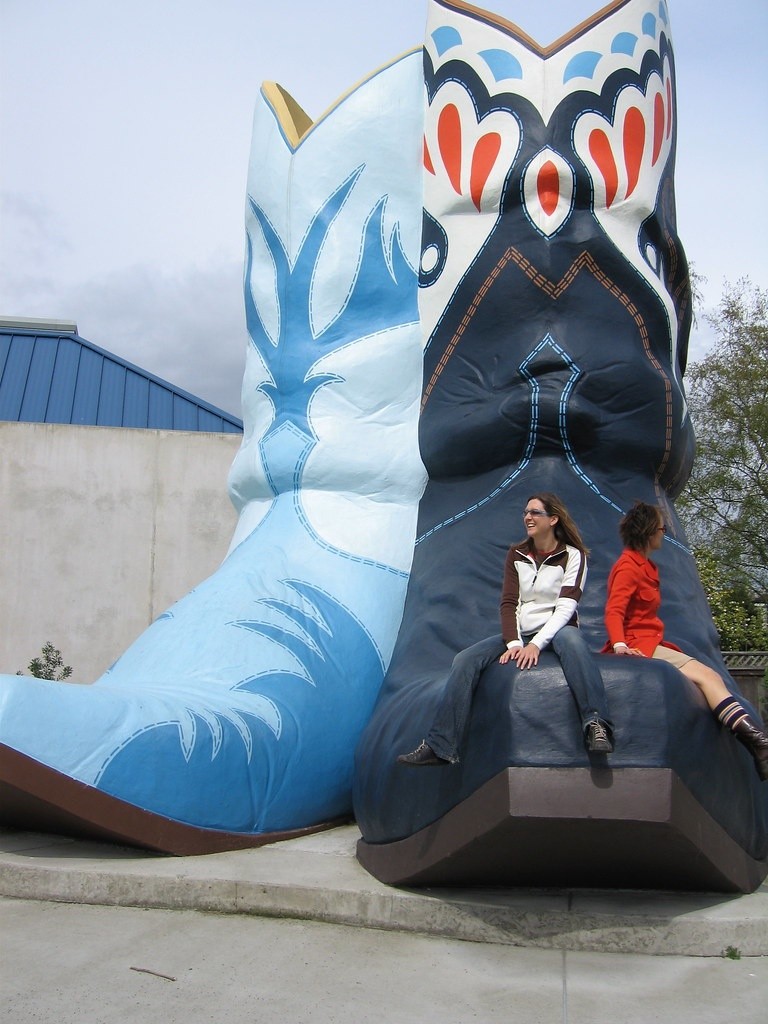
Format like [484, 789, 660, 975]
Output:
[659, 525, 666, 533]
[522, 509, 546, 517]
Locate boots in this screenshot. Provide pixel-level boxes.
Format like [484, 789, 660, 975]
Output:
[732, 717, 768, 781]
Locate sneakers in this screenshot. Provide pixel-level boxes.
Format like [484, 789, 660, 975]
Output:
[586, 722, 613, 753]
[399, 743, 449, 768]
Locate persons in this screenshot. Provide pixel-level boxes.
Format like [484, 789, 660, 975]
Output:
[603, 501, 768, 780]
[397, 489, 614, 765]
